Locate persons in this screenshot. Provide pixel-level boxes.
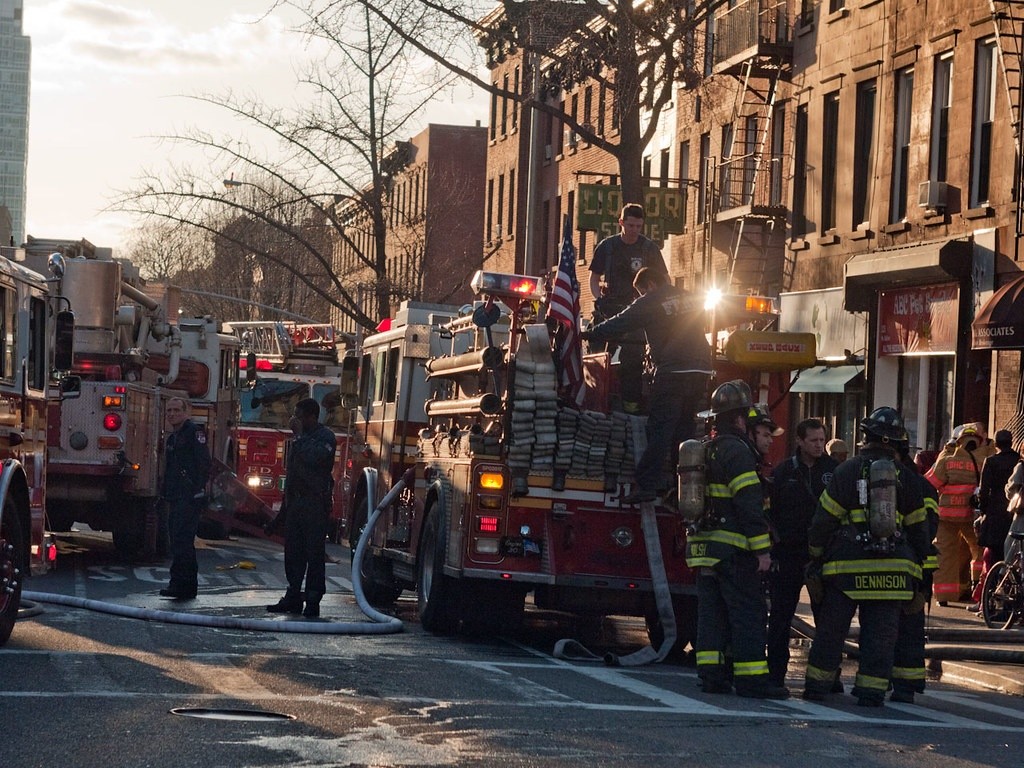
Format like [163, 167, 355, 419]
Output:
[925, 422, 1024, 616]
[265, 399, 335, 617]
[582, 270, 709, 505]
[589, 203, 672, 414]
[687, 380, 939, 705]
[160, 397, 213, 596]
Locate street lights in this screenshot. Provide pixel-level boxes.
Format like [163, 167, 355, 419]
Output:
[221, 179, 294, 311]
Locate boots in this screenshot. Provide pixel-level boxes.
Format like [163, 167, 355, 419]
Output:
[266, 586, 304, 614]
[303, 589, 323, 616]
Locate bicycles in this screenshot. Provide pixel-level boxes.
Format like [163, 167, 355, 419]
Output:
[980, 531, 1024, 630]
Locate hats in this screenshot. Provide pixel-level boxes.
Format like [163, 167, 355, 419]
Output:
[825, 439, 849, 456]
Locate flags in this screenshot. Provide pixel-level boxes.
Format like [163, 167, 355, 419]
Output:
[544, 218, 587, 385]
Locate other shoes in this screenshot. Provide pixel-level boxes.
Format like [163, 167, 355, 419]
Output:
[857, 696, 885, 708]
[939, 600, 947, 606]
[622, 489, 657, 504]
[833, 667, 844, 693]
[701, 676, 734, 694]
[736, 685, 791, 700]
[966, 602, 980, 612]
[159, 580, 197, 597]
[802, 689, 824, 700]
[889, 689, 915, 704]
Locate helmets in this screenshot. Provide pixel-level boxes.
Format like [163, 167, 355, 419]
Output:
[746, 403, 777, 433]
[955, 427, 983, 445]
[859, 406, 908, 441]
[711, 380, 753, 413]
[946, 425, 964, 445]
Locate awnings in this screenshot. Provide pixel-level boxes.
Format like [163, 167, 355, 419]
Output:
[844, 240, 972, 313]
[788, 365, 866, 392]
[971, 275, 1024, 350]
[708, 294, 779, 326]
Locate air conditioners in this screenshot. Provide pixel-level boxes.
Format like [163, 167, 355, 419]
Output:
[918, 181, 947, 207]
[576, 123, 590, 141]
[564, 130, 578, 147]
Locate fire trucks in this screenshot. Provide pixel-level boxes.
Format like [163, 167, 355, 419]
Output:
[0, 206, 354, 653]
[322, 270, 819, 656]
